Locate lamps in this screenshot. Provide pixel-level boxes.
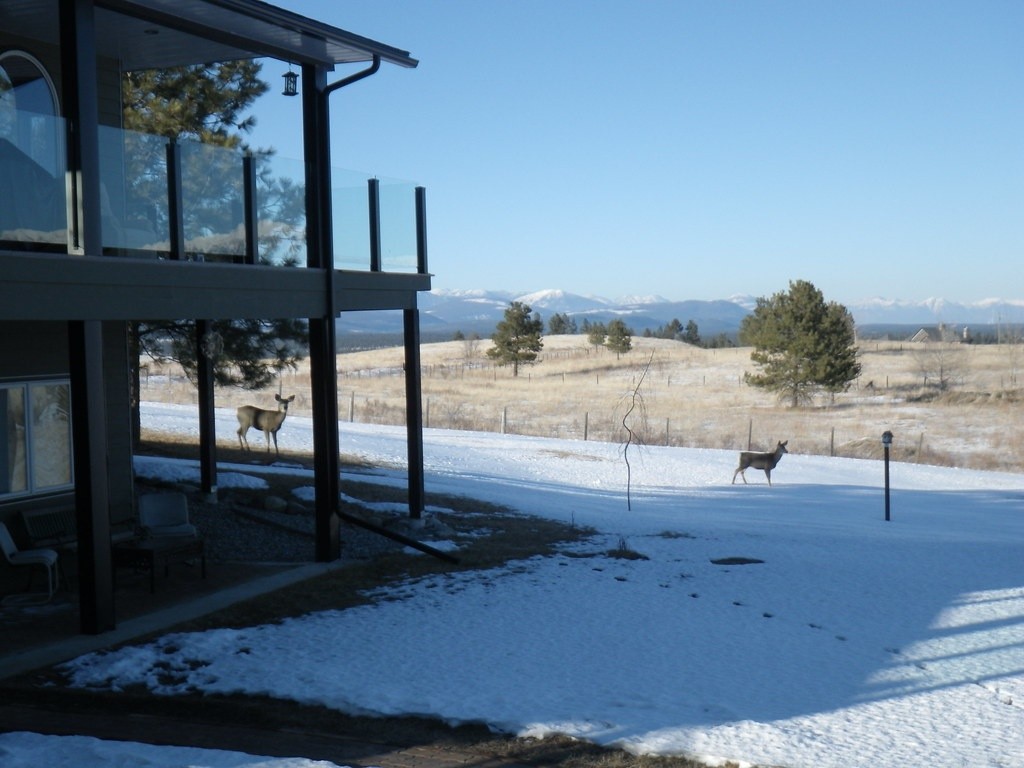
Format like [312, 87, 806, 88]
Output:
[282, 63, 298, 96]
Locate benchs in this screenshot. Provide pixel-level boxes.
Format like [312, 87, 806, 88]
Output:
[20, 507, 134, 584]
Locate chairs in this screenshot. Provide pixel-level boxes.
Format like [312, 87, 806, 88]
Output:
[0, 523, 59, 606]
[139, 492, 206, 593]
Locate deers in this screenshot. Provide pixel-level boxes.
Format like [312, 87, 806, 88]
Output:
[731, 440, 788, 486]
[235, 393, 295, 455]
